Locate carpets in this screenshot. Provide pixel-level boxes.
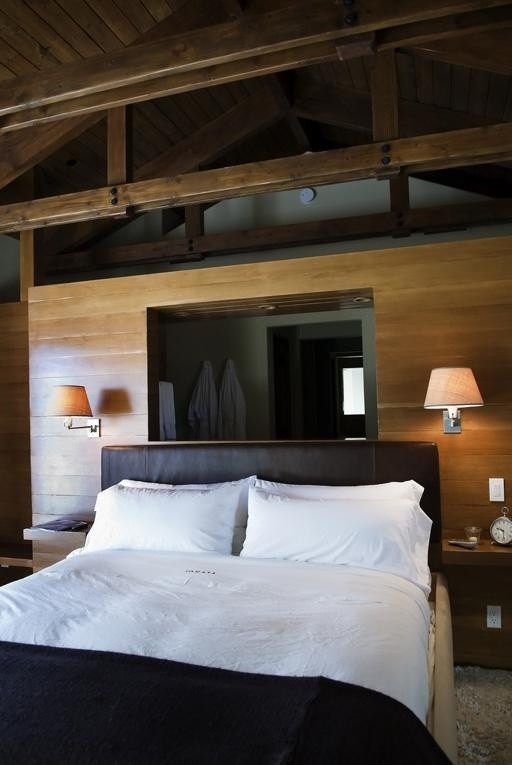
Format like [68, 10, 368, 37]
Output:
[454, 664, 512, 765]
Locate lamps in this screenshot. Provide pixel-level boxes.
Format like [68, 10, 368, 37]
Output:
[424, 369, 483, 433]
[48, 386, 100, 438]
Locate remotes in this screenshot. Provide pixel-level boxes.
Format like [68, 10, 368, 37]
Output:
[448, 540, 475, 548]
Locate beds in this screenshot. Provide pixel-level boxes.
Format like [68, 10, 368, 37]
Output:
[1, 442, 456, 764]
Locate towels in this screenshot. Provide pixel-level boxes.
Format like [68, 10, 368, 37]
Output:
[158, 381, 176, 441]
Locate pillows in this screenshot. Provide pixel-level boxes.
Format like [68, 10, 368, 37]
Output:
[84, 485, 241, 555]
[254, 478, 425, 505]
[239, 485, 431, 590]
[118, 476, 256, 555]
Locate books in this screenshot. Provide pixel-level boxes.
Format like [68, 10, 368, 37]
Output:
[33, 518, 89, 532]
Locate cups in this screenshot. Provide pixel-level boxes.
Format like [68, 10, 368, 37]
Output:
[462, 526, 482, 545]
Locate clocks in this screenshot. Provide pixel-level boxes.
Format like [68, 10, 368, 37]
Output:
[490, 508, 512, 545]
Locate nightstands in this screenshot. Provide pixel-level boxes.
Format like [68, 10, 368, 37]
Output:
[442, 540, 512, 670]
[23, 525, 91, 573]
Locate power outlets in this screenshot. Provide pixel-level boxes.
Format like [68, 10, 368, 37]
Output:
[486, 605, 501, 628]
